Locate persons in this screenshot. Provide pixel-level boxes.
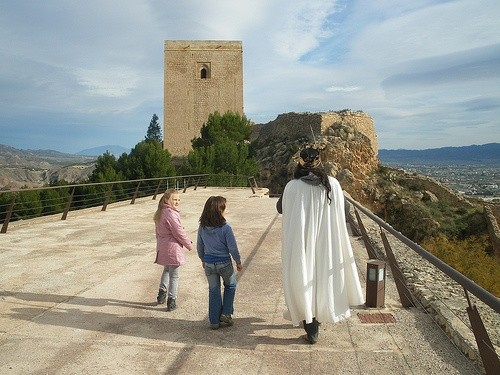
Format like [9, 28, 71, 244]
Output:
[276, 148, 366, 344]
[196, 196, 243, 330]
[152, 188, 195, 312]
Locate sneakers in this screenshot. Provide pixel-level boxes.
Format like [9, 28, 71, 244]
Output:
[211, 323, 221, 329]
[220, 314, 234, 324]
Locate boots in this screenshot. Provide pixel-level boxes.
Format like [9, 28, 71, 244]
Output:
[303, 317, 321, 343]
[167, 298, 177, 311]
[157, 289, 167, 304]
